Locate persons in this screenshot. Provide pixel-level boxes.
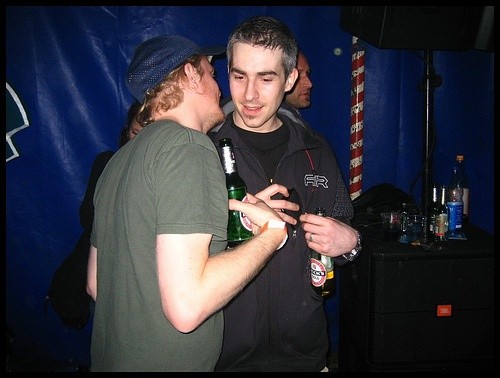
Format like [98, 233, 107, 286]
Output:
[79, 101, 145, 318]
[85, 33, 286, 372]
[205, 16, 362, 372]
[277, 49, 312, 135]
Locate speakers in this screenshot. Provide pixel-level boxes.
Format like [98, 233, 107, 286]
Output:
[341, 5, 486, 53]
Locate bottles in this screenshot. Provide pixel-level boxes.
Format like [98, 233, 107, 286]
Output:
[444, 167, 465, 239]
[455, 155, 471, 226]
[428, 184, 439, 243]
[219, 137, 254, 251]
[434, 185, 450, 243]
[310, 208, 336, 298]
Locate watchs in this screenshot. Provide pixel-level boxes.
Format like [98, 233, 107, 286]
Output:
[343, 231, 362, 261]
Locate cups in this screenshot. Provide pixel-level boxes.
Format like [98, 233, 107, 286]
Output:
[381, 211, 403, 242]
[403, 215, 427, 246]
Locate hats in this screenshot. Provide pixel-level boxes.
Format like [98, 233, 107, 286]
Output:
[126, 34, 227, 105]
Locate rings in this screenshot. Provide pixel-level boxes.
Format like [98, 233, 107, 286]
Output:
[309, 233, 314, 242]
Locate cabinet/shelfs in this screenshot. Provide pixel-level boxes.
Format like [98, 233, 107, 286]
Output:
[340, 219, 500, 378]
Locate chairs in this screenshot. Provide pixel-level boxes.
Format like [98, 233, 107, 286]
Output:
[351, 184, 417, 219]
